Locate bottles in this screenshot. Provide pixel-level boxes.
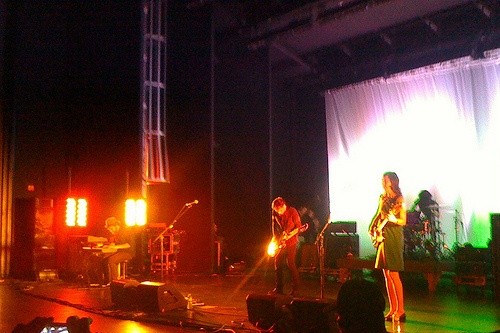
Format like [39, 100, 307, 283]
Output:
[186, 293, 193, 309]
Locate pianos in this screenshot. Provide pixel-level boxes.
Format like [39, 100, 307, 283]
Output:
[79, 243, 118, 254]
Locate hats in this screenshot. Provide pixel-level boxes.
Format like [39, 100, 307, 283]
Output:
[104, 217, 121, 228]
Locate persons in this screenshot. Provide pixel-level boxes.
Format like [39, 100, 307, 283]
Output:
[369, 172, 407, 322]
[410, 190, 439, 244]
[89, 216, 136, 287]
[266, 197, 303, 296]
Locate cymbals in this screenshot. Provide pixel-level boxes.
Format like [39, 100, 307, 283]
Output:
[424, 205, 453, 209]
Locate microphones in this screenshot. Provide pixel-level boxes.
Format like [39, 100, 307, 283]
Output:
[186, 200, 199, 206]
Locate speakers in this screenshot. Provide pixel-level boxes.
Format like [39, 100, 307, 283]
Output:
[299, 234, 359, 269]
[110, 279, 189, 313]
[245, 293, 339, 333]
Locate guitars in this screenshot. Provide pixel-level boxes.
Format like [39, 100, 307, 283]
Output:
[371, 199, 404, 248]
[266, 223, 309, 258]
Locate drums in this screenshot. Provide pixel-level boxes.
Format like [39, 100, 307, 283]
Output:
[405, 210, 420, 226]
[414, 219, 430, 232]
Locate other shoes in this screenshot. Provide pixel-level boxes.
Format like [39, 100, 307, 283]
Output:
[290, 286, 305, 291]
[90, 280, 100, 287]
[388, 312, 406, 322]
[268, 288, 283, 295]
[384, 313, 393, 321]
[101, 282, 110, 288]
